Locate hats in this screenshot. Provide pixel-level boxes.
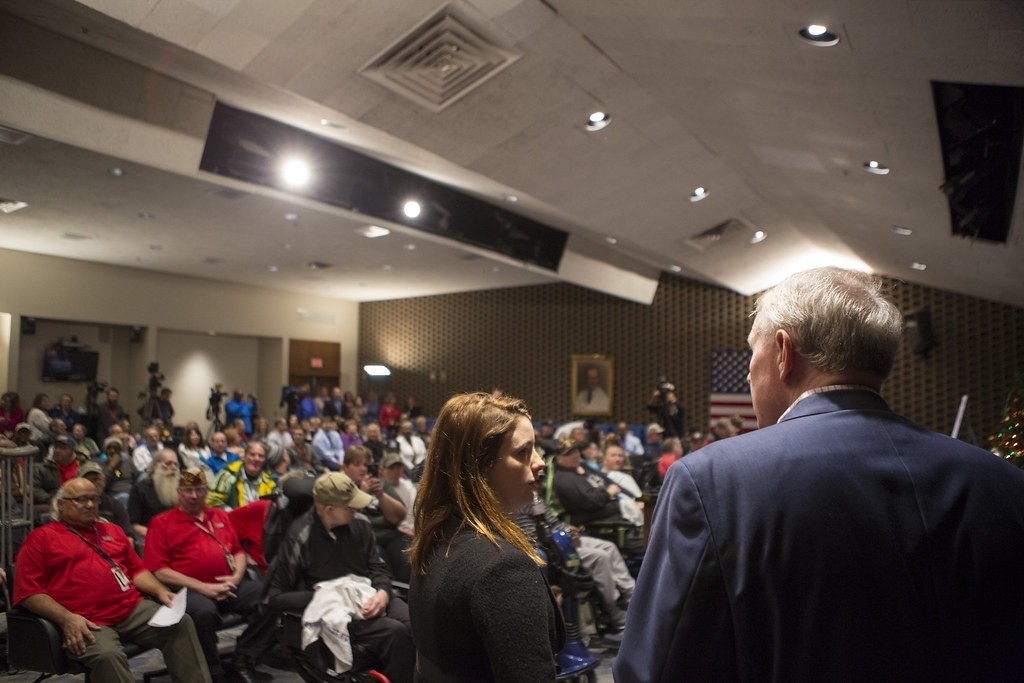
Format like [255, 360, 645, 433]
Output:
[79, 461, 103, 477]
[384, 453, 404, 468]
[104, 436, 122, 450]
[561, 446, 577, 455]
[647, 423, 665, 434]
[178, 467, 208, 487]
[15, 422, 31, 432]
[55, 435, 76, 447]
[311, 472, 372, 510]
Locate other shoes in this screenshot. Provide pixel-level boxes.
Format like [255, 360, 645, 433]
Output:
[609, 589, 632, 629]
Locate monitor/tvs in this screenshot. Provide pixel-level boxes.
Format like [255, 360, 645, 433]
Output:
[42, 349, 99, 383]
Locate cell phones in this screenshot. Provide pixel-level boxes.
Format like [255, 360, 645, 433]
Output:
[367, 464, 379, 478]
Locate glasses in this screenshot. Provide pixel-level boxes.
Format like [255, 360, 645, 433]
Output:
[62, 494, 100, 504]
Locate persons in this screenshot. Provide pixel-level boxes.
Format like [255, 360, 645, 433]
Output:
[610, 265, 1024, 683]
[0, 382, 746, 683]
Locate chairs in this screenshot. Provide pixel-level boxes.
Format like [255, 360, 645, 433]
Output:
[5, 520, 629, 683]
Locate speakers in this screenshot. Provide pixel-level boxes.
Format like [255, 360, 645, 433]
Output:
[901, 309, 933, 356]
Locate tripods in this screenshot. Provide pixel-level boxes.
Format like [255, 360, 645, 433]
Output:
[205, 403, 224, 439]
[144, 390, 162, 427]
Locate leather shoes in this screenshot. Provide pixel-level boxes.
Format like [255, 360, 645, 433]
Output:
[230, 658, 260, 683]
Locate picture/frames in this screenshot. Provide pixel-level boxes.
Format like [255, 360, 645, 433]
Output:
[570, 354, 613, 416]
